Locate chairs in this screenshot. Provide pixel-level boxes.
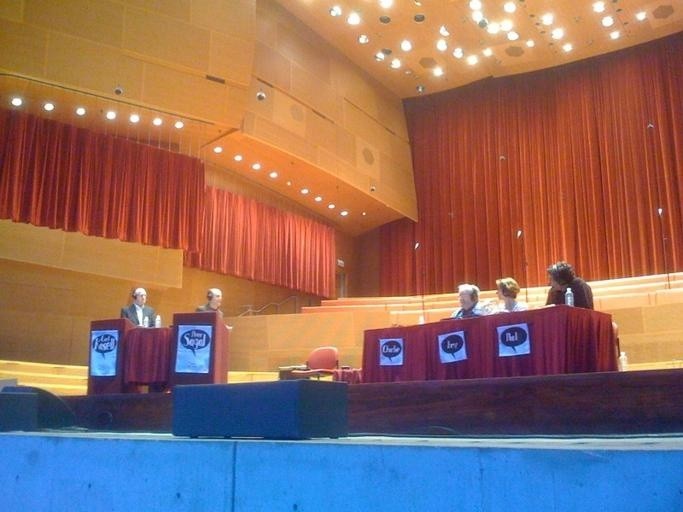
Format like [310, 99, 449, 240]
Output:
[291, 347, 338, 381]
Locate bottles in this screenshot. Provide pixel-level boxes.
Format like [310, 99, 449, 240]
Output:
[564, 288, 574, 306]
[155, 316, 161, 328]
[619, 352, 627, 371]
[144, 317, 149, 327]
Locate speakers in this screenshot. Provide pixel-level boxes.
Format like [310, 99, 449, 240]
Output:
[0, 385, 78, 432]
[172, 379, 349, 440]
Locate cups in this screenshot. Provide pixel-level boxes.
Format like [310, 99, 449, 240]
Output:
[419, 316, 425, 325]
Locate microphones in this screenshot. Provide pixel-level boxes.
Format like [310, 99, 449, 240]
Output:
[516, 230, 522, 237]
[414, 242, 420, 249]
[658, 208, 662, 215]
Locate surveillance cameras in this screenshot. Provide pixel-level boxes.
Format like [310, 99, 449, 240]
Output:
[256, 92, 265, 100]
[114, 88, 122, 95]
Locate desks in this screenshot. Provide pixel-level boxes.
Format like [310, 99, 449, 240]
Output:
[363, 305, 618, 383]
[87, 311, 229, 393]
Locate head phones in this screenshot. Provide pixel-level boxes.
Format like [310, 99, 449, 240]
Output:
[553, 264, 567, 285]
[132, 292, 137, 299]
[501, 279, 511, 296]
[207, 292, 213, 300]
[471, 288, 478, 302]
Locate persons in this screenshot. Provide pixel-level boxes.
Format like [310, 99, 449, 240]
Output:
[196, 288, 224, 320]
[449, 261, 594, 319]
[121, 287, 156, 328]
